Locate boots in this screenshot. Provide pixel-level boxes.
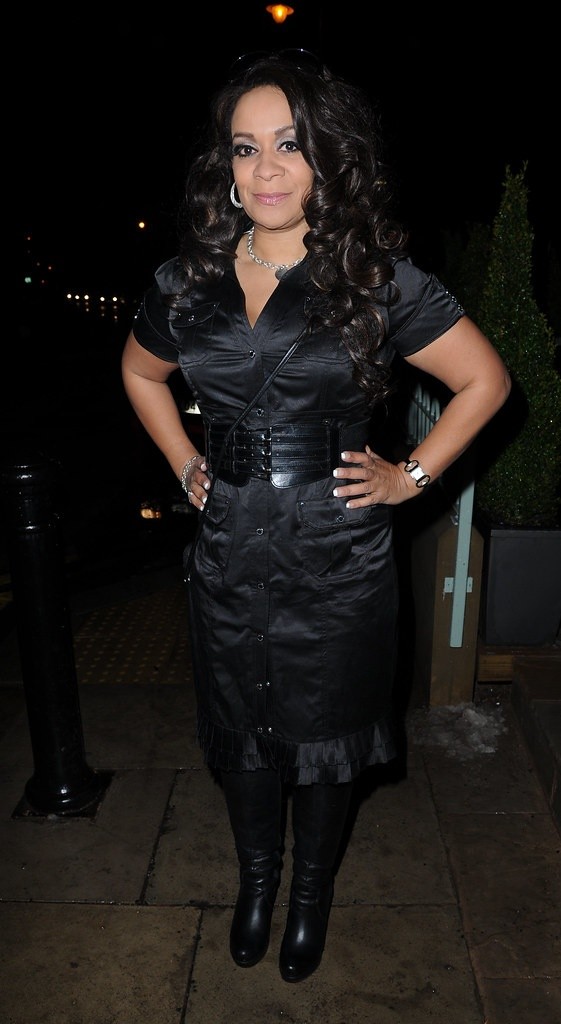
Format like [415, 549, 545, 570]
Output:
[279, 780, 352, 984]
[219, 771, 288, 968]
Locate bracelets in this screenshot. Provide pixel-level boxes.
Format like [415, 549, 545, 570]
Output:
[181, 456, 198, 493]
[400, 454, 430, 490]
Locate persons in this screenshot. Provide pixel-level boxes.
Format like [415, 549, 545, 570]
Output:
[122, 48, 513, 983]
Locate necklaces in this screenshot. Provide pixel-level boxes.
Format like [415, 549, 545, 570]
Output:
[247, 227, 303, 271]
